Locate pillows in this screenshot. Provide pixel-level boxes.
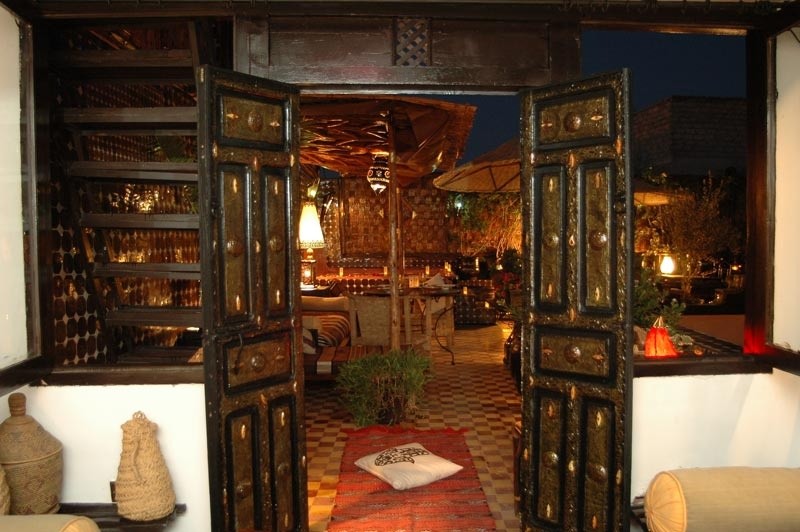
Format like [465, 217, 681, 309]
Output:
[353, 442, 464, 492]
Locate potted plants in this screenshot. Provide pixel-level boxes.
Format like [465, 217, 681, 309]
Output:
[330, 344, 435, 427]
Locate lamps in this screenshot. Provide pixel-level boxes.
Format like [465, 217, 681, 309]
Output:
[643, 316, 679, 358]
[366, 153, 390, 198]
[296, 201, 325, 288]
[654, 253, 677, 275]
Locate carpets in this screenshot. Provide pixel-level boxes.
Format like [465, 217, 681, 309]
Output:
[324, 426, 498, 532]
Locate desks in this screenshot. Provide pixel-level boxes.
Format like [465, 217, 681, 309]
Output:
[661, 275, 728, 303]
[363, 288, 462, 365]
